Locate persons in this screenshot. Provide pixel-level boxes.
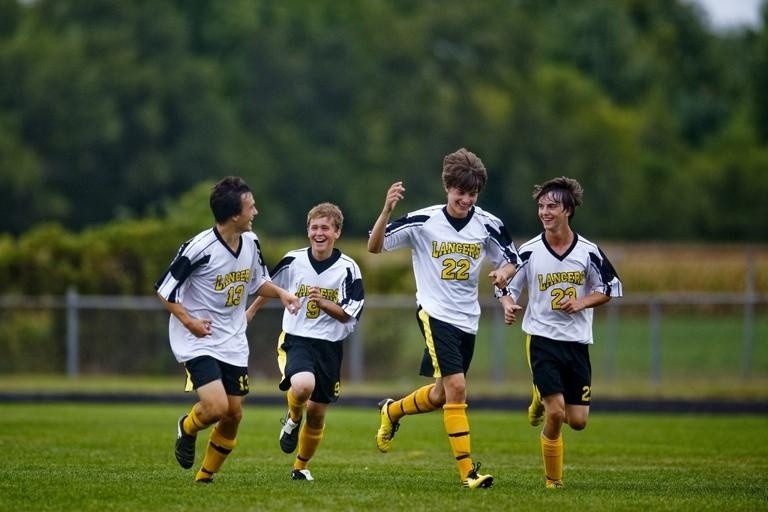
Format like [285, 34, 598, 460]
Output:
[246, 203, 363, 482]
[494, 177, 623, 487]
[153, 176, 298, 483]
[367, 147, 521, 489]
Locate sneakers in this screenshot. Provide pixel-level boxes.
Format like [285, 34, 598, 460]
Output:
[279, 411, 301, 454]
[546, 479, 565, 490]
[463, 470, 493, 488]
[175, 415, 196, 470]
[376, 399, 399, 453]
[291, 469, 314, 480]
[528, 384, 545, 426]
